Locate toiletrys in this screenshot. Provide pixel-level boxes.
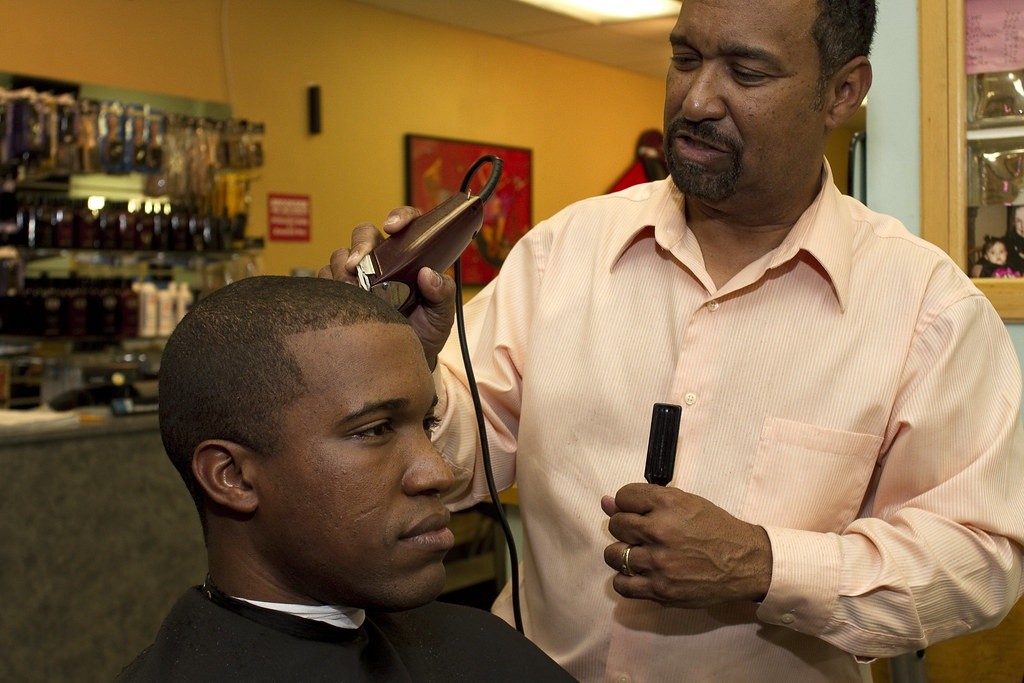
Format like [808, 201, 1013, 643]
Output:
[137, 275, 197, 343]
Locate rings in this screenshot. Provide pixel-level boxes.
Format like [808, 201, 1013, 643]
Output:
[618, 543, 638, 577]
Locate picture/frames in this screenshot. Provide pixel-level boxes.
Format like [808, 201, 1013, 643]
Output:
[403, 129, 533, 286]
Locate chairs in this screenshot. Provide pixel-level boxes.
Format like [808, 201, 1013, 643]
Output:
[969, 244, 986, 267]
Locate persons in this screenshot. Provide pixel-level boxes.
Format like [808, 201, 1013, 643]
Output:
[978, 234, 1021, 278]
[111, 273, 579, 683]
[316, 0, 1024, 683]
[970, 206, 1024, 279]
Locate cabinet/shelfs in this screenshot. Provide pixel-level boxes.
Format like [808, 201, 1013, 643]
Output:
[1, 85, 269, 411]
